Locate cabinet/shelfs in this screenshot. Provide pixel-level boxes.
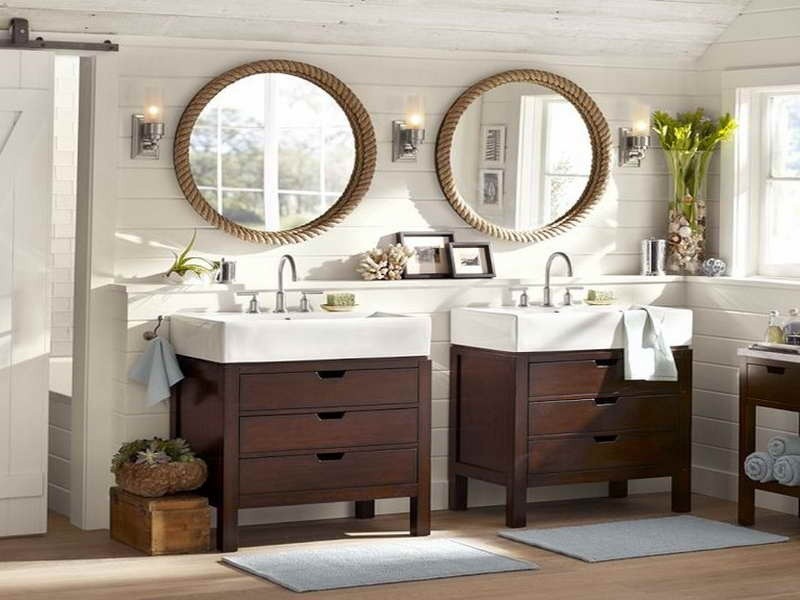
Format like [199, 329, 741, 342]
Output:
[448, 347, 693, 528]
[170, 354, 432, 552]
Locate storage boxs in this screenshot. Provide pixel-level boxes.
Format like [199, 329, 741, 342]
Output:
[109, 486, 212, 556]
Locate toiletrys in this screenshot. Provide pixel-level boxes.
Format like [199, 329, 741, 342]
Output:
[763, 308, 800, 345]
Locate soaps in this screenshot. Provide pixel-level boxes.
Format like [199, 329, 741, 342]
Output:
[587, 288, 616, 301]
[326, 292, 356, 306]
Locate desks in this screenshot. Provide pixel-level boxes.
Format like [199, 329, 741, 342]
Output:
[736, 349, 800, 527]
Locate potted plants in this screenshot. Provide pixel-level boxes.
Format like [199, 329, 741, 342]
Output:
[646, 108, 739, 276]
[320, 292, 360, 312]
[161, 228, 220, 284]
[585, 289, 617, 306]
[111, 435, 208, 497]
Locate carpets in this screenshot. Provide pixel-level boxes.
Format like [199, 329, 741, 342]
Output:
[221, 537, 542, 593]
[496, 515, 790, 564]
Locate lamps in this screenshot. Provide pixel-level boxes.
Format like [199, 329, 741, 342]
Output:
[391, 94, 425, 164]
[131, 86, 167, 161]
[618, 103, 652, 167]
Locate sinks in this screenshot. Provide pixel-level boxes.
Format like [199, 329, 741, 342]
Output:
[168, 308, 433, 363]
[448, 303, 694, 351]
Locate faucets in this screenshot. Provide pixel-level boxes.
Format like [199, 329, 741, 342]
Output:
[543, 251, 574, 306]
[272, 253, 297, 312]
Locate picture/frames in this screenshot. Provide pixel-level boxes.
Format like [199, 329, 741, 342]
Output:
[395, 230, 455, 279]
[446, 242, 496, 280]
[480, 124, 506, 169]
[478, 169, 505, 210]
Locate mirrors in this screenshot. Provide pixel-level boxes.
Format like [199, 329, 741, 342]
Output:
[435, 69, 613, 244]
[173, 58, 379, 247]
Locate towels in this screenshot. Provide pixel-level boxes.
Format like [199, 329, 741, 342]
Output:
[618, 303, 679, 382]
[743, 433, 800, 486]
[128, 336, 185, 409]
